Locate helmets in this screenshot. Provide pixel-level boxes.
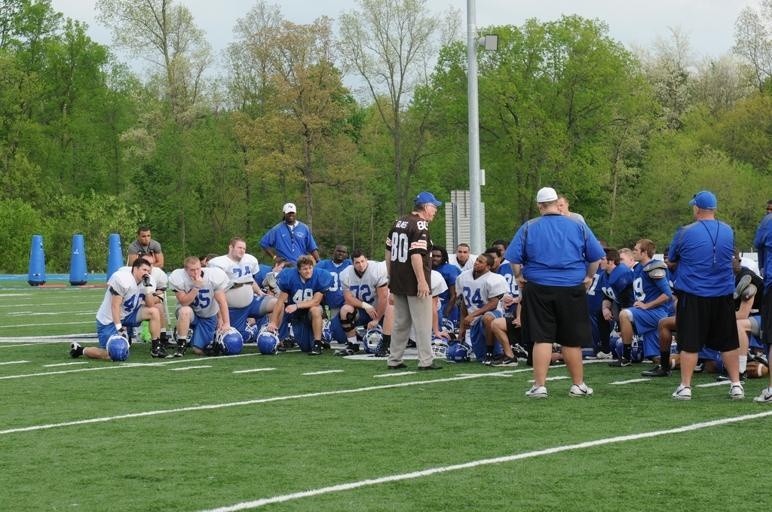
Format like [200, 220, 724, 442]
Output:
[106, 335, 130, 360]
[431, 318, 473, 362]
[216, 324, 280, 353]
[321, 318, 333, 343]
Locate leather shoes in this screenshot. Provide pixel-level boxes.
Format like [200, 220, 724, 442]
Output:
[418, 363, 442, 370]
[389, 363, 407, 369]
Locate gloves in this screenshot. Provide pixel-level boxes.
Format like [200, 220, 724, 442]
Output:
[142, 274, 152, 287]
[204, 341, 220, 356]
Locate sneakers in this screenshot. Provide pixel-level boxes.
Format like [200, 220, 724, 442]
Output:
[151, 332, 187, 358]
[71, 342, 81, 359]
[527, 386, 548, 397]
[281, 338, 360, 356]
[483, 343, 528, 366]
[528, 352, 772, 406]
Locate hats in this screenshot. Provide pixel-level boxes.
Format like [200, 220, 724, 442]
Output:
[415, 193, 442, 207]
[689, 191, 717, 210]
[537, 187, 558, 203]
[283, 203, 296, 215]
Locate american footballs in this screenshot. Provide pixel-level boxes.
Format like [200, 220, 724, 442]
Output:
[670, 353, 681, 369]
[746, 361, 769, 378]
